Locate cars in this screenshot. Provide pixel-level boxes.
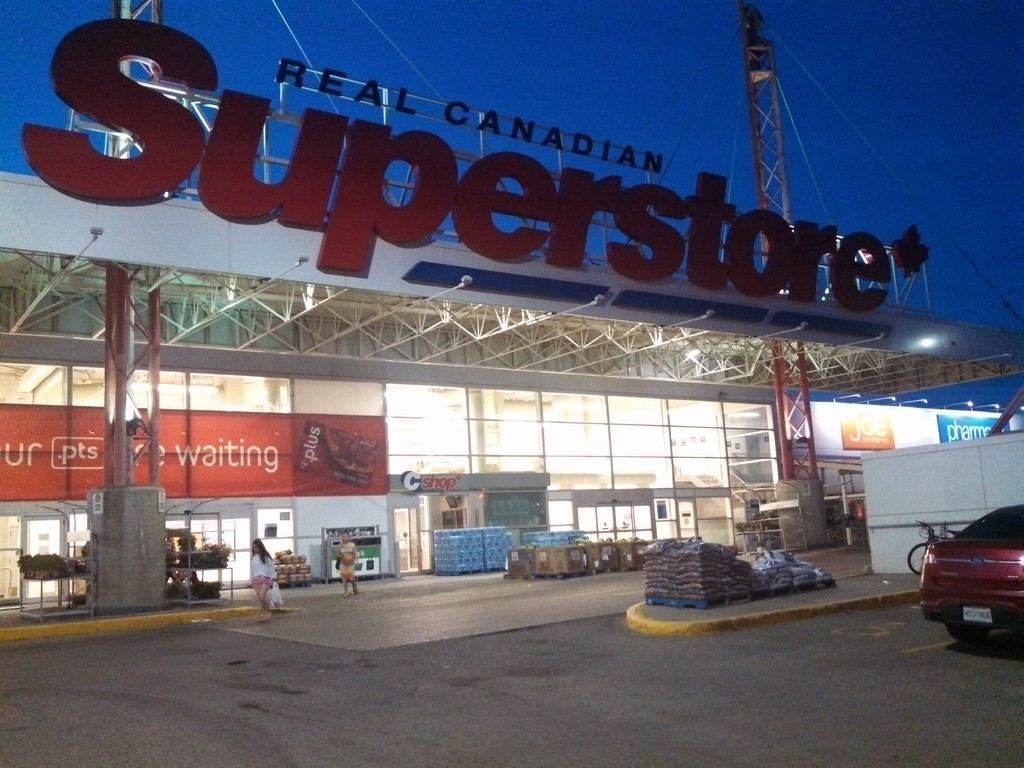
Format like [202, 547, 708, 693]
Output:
[920, 504, 1024, 642]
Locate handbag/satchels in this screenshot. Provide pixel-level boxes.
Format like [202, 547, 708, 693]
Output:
[271, 581, 282, 608]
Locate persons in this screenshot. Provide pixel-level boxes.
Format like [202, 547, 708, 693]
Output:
[335, 533, 359, 598]
[248, 538, 279, 621]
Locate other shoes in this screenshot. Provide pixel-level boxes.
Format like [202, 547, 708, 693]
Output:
[354, 591, 358, 595]
[263, 614, 272, 621]
[344, 593, 349, 597]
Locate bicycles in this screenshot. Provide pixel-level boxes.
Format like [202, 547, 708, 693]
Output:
[907, 519, 960, 576]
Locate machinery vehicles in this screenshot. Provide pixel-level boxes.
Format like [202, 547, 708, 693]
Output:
[740, 0, 823, 480]
